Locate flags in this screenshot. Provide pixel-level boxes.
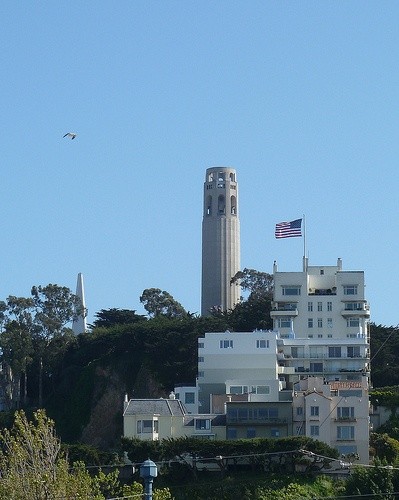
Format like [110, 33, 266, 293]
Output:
[275, 218, 303, 239]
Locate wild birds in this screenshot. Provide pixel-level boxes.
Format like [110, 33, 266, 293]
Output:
[63, 132, 77, 140]
